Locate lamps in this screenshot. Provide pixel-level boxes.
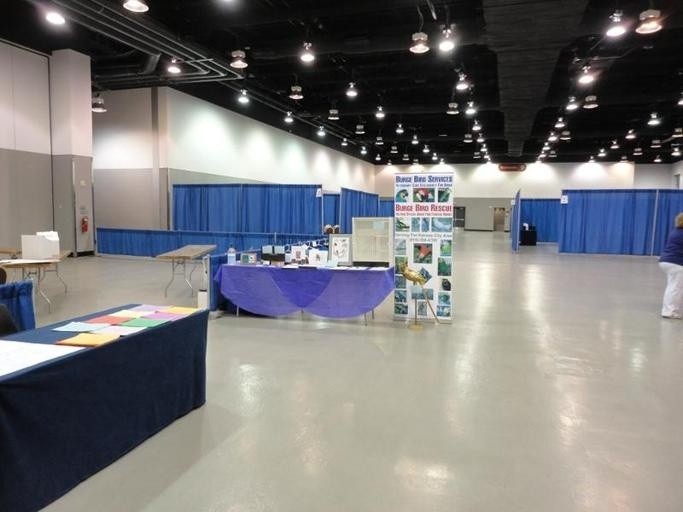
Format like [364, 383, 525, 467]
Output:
[168, 58, 181, 74]
[410, 1, 429, 53]
[583, 95, 598, 110]
[230, 51, 248, 68]
[300, 42, 315, 63]
[123, 1, 149, 12]
[438, 6, 454, 52]
[588, 129, 682, 165]
[376, 124, 445, 165]
[578, 62, 594, 84]
[283, 111, 293, 122]
[317, 126, 325, 137]
[328, 110, 341, 121]
[346, 82, 359, 99]
[606, 8, 626, 36]
[447, 92, 460, 115]
[635, 10, 663, 35]
[355, 125, 365, 135]
[92, 93, 107, 112]
[238, 87, 249, 104]
[376, 106, 385, 119]
[565, 96, 578, 111]
[360, 147, 367, 156]
[648, 111, 660, 126]
[341, 137, 349, 148]
[536, 117, 571, 165]
[289, 85, 303, 100]
[456, 73, 469, 91]
[463, 93, 492, 164]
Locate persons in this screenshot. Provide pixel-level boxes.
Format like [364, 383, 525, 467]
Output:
[657, 212, 683, 319]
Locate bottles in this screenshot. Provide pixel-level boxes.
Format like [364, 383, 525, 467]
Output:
[227, 243, 236, 264]
[197, 289, 207, 310]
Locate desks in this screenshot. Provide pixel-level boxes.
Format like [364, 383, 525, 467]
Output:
[0, 303, 209, 510]
[156, 244, 216, 300]
[0, 247, 72, 315]
[212, 262, 395, 318]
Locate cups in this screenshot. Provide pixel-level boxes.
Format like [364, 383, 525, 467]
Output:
[284, 254, 291, 263]
[240, 254, 247, 264]
